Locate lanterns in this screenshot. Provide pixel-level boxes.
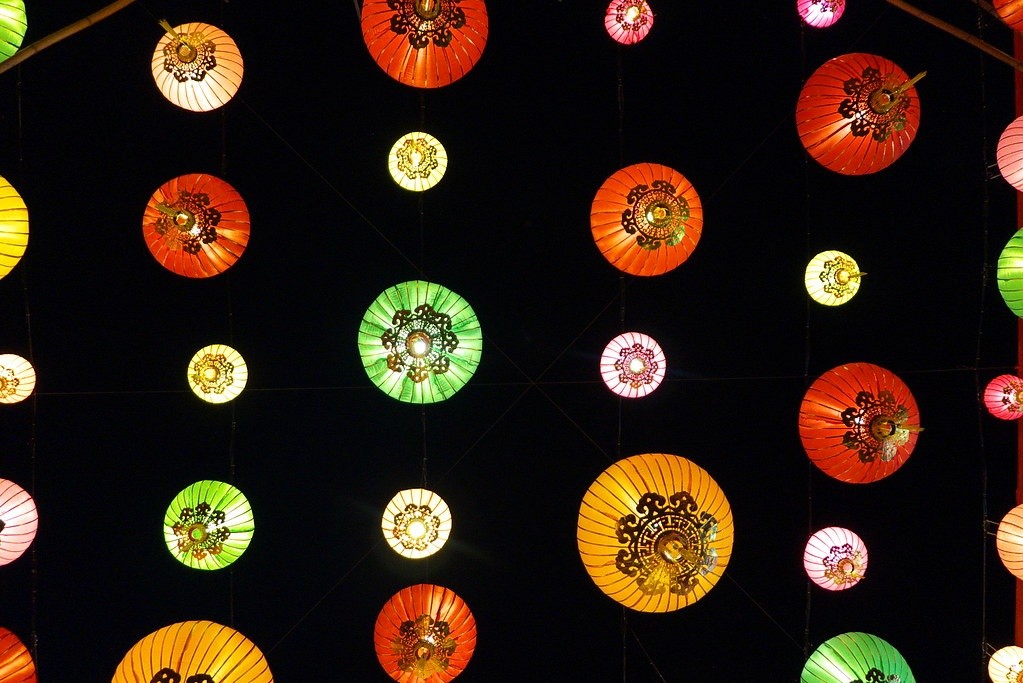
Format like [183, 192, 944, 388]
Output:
[603, 0, 654, 44]
[187, 344, 250, 405]
[163, 480, 255, 571]
[806, 251, 867, 307]
[142, 171, 250, 277]
[0, 628, 38, 683]
[109, 620, 275, 682]
[799, 632, 916, 683]
[381, 488, 451, 559]
[796, 0, 848, 28]
[357, 280, 484, 404]
[794, 53, 928, 176]
[0, 354, 36, 407]
[804, 528, 870, 593]
[598, 332, 666, 397]
[387, 131, 448, 192]
[0, 0, 27, 63]
[983, 375, 1023, 421]
[577, 454, 735, 614]
[994, 1, 1022, 325]
[362, 0, 488, 87]
[0, 478, 37, 565]
[150, 17, 244, 114]
[374, 584, 477, 683]
[997, 502, 1023, 581]
[0, 176, 31, 281]
[590, 162, 704, 277]
[799, 362, 924, 484]
[988, 645, 1023, 683]
